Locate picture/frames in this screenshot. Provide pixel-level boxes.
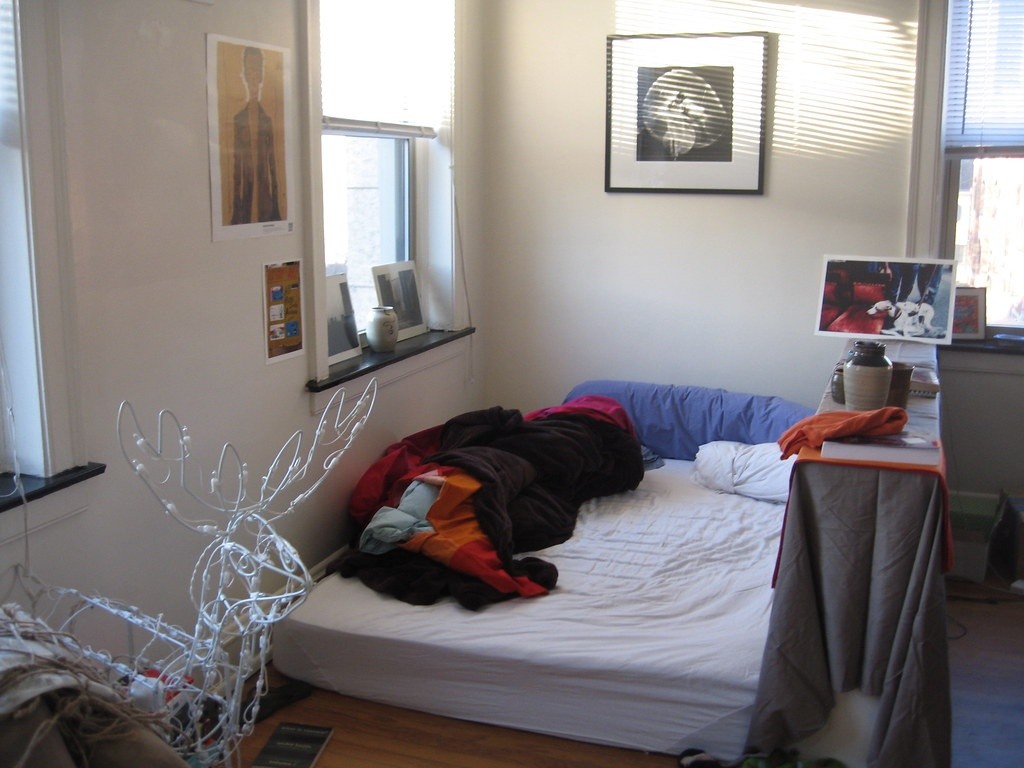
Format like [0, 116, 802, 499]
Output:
[206, 33, 295, 242]
[605, 31, 769, 195]
[325, 273, 362, 366]
[952, 287, 987, 342]
[372, 260, 428, 343]
[814, 255, 958, 347]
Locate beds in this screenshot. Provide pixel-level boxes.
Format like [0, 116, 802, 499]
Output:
[272, 380, 824, 766]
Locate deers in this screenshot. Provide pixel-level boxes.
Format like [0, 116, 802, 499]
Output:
[2, 377, 379, 768]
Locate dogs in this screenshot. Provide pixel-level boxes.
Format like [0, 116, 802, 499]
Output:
[865, 299, 946, 338]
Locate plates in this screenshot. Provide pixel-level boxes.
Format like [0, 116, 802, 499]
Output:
[993, 333, 1024, 347]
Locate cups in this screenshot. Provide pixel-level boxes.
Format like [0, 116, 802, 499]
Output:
[831, 369, 846, 405]
[885, 361, 916, 410]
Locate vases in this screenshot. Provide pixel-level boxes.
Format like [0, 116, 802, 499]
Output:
[365, 307, 399, 352]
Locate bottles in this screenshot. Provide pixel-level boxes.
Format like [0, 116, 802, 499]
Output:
[843, 341, 893, 411]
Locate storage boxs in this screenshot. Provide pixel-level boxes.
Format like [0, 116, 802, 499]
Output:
[945, 486, 1024, 585]
